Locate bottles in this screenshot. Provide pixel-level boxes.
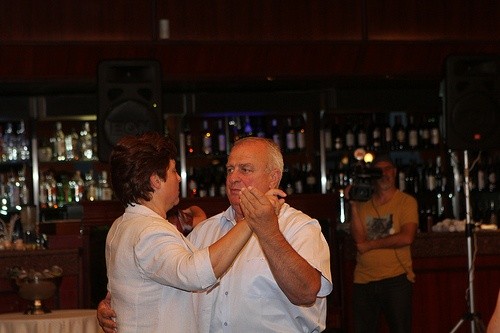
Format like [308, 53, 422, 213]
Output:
[179, 94, 500, 236]
[0, 92, 115, 252]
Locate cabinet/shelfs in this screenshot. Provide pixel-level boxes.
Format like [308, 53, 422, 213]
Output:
[0, 83, 500, 241]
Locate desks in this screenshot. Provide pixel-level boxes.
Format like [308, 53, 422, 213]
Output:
[0, 309, 104, 333]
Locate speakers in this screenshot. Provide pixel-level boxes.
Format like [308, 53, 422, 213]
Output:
[97, 59, 162, 163]
[444, 52, 500, 151]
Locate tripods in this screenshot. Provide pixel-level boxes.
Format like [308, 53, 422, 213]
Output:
[449, 150, 487, 333]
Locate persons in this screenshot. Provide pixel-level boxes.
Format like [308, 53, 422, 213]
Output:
[104, 130, 285, 333]
[345, 155, 418, 333]
[97, 136, 333, 333]
